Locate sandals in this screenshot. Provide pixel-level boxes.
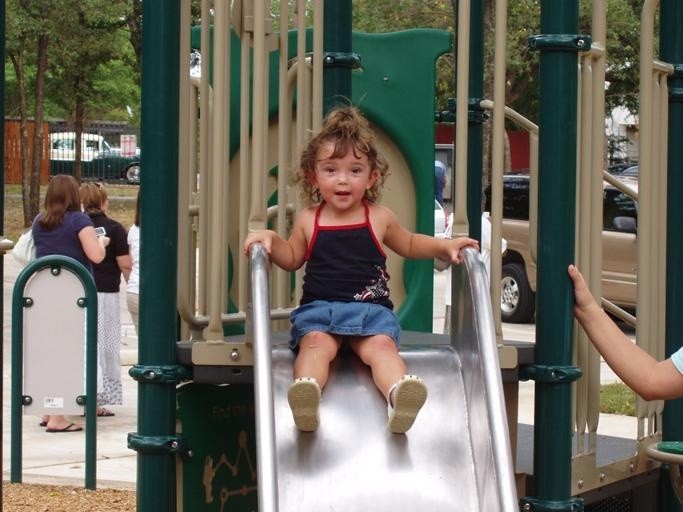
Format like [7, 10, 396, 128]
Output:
[288, 377, 320, 432]
[388, 375, 428, 434]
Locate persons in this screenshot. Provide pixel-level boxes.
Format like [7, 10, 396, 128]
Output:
[565, 262, 682, 402]
[63, 180, 133, 421]
[241, 96, 480, 436]
[125, 185, 140, 338]
[29, 171, 111, 434]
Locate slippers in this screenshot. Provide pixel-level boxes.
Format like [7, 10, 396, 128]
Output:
[40, 421, 82, 432]
[80, 408, 114, 417]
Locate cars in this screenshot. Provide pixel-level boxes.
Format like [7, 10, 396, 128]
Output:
[435, 199, 450, 241]
[92, 147, 142, 185]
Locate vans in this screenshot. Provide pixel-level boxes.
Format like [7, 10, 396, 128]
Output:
[479, 171, 640, 327]
[48, 132, 121, 165]
[435, 159, 447, 206]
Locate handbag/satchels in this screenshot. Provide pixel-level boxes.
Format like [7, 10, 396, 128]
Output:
[11, 229, 36, 264]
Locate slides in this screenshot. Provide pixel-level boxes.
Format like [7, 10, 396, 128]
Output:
[251, 235, 520, 512]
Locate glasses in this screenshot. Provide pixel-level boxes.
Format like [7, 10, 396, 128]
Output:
[80, 182, 100, 190]
